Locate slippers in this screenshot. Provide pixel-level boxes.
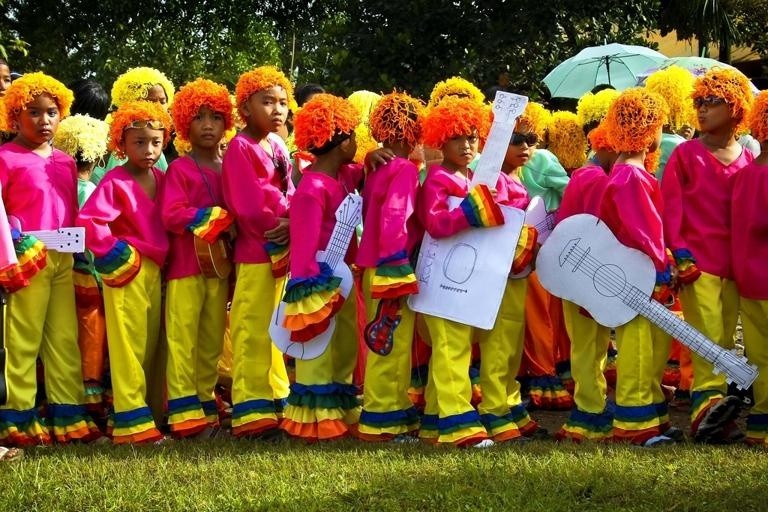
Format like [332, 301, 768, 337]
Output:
[0, 446, 24, 464]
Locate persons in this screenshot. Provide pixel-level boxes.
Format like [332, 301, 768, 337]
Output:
[2, 59, 767, 461]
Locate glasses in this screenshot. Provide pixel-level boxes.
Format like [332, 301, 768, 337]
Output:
[694, 95, 724, 107]
[509, 132, 539, 146]
[123, 119, 166, 131]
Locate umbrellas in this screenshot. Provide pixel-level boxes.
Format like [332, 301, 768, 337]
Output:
[635, 45, 761, 97]
[541, 36, 670, 101]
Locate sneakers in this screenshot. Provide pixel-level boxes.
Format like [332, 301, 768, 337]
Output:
[471, 426, 549, 449]
[695, 395, 746, 444]
[88, 414, 231, 448]
[644, 427, 684, 447]
[390, 434, 420, 446]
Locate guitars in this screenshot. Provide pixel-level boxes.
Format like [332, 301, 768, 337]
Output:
[407, 89, 527, 331]
[23, 225, 87, 255]
[269, 187, 364, 362]
[192, 230, 234, 283]
[363, 239, 423, 357]
[536, 214, 761, 394]
[508, 195, 557, 280]
